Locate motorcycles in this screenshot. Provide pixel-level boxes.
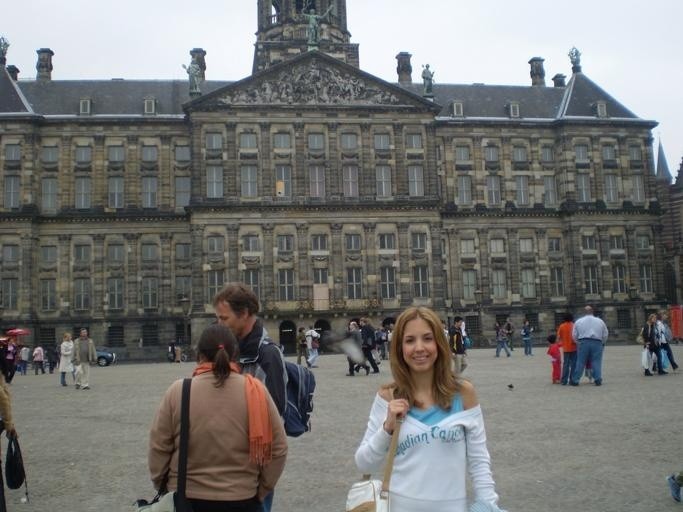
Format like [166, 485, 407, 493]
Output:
[166, 346, 189, 362]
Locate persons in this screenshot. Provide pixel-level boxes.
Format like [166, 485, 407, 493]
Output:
[421, 63, 434, 93]
[181, 57, 201, 92]
[300, 1, 335, 46]
[211, 281, 289, 511]
[441, 316, 469, 374]
[296, 325, 319, 368]
[146, 323, 287, 512]
[0, 375, 19, 512]
[169, 339, 180, 363]
[495, 315, 534, 358]
[642, 309, 680, 376]
[352, 307, 506, 512]
[547, 313, 609, 386]
[1, 327, 98, 390]
[664, 469, 682, 504]
[346, 317, 393, 376]
[567, 45, 582, 66]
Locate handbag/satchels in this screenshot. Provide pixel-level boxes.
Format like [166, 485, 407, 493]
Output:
[130, 487, 185, 512]
[342, 383, 403, 512]
[634, 323, 650, 346]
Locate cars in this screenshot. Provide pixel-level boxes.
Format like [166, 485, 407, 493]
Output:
[53, 345, 117, 366]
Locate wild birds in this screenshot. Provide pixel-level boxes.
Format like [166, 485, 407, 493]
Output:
[317, 330, 367, 366]
[507, 383, 514, 390]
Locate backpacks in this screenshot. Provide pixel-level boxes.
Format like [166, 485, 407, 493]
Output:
[253, 334, 316, 438]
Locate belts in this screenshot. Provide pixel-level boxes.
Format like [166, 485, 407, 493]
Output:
[575, 337, 603, 343]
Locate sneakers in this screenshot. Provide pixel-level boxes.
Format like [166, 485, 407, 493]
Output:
[657, 369, 669, 375]
[643, 368, 652, 376]
[550, 378, 603, 387]
[61, 383, 91, 391]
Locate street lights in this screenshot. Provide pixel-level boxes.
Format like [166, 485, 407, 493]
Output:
[180, 294, 193, 361]
[470, 288, 485, 347]
